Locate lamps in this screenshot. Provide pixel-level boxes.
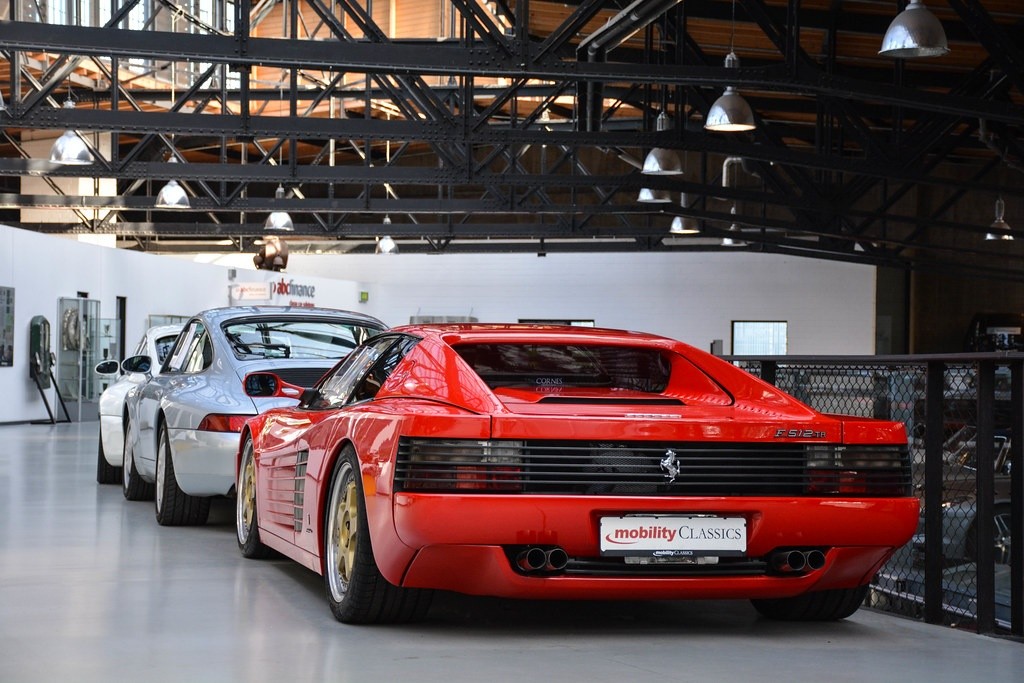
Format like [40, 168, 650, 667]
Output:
[47, 0, 954, 272]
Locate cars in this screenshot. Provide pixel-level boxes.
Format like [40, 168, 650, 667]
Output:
[121, 305, 390, 526]
[890, 326, 1012, 624]
[92, 323, 216, 485]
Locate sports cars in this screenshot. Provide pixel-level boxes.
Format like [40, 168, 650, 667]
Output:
[236, 323, 919, 630]
